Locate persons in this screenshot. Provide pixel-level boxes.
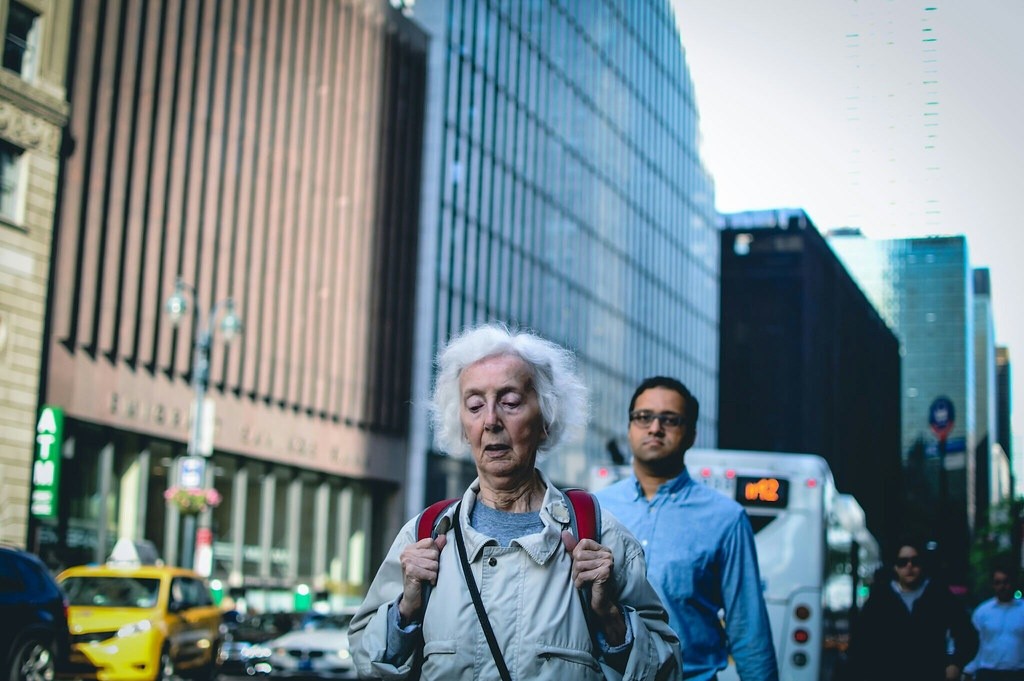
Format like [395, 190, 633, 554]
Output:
[347, 325, 685, 681]
[853, 542, 979, 681]
[588, 376, 780, 681]
[945, 566, 1024, 681]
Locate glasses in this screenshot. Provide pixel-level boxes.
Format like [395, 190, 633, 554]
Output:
[631, 410, 683, 429]
[894, 556, 921, 566]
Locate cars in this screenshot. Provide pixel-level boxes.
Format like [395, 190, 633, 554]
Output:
[55, 531, 225, 680]
[0, 543, 71, 681]
[219, 612, 307, 676]
[263, 606, 359, 679]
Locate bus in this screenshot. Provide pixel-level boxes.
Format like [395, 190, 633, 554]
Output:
[587, 448, 883, 681]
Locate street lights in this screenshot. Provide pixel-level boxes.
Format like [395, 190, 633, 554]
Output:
[164, 275, 244, 561]
